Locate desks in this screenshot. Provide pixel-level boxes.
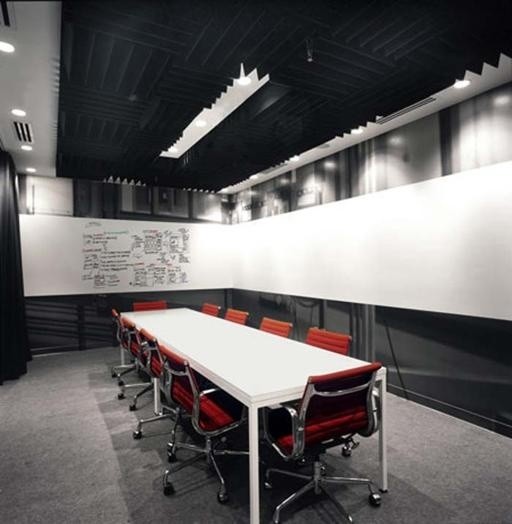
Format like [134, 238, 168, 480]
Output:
[117, 308, 388, 524]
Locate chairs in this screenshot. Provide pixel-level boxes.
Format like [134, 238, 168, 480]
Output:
[132, 328, 167, 440]
[224, 308, 249, 324]
[117, 315, 151, 410]
[156, 343, 249, 504]
[256, 317, 294, 337]
[110, 311, 122, 318]
[132, 300, 168, 311]
[261, 360, 383, 524]
[306, 326, 351, 356]
